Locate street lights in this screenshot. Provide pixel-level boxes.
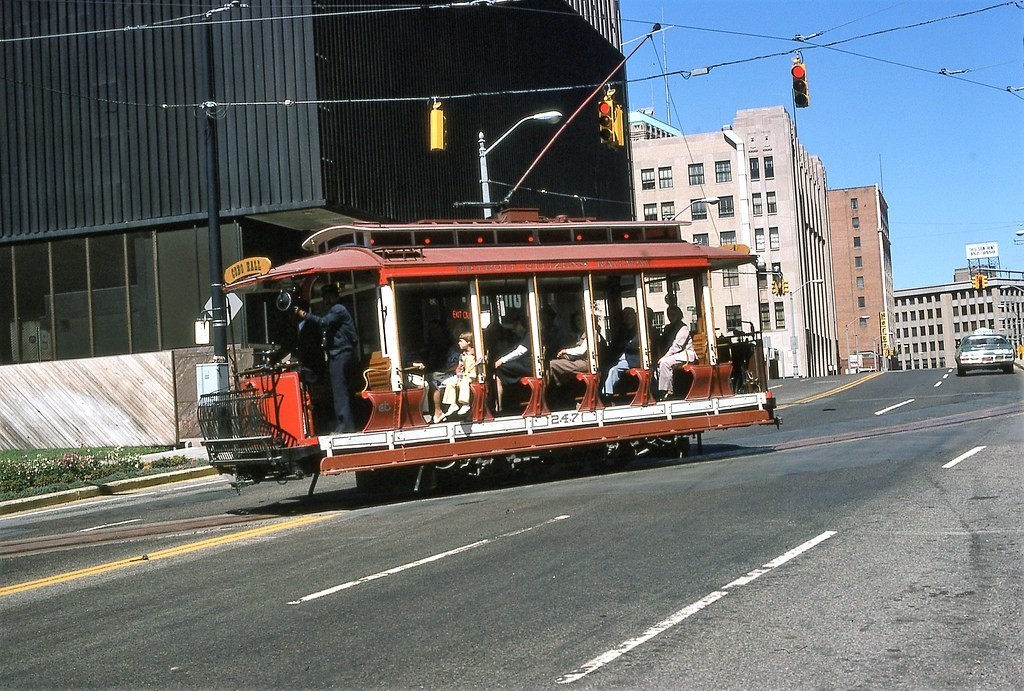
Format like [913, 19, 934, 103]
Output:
[478, 110, 563, 316]
[845, 315, 871, 374]
[789, 279, 824, 379]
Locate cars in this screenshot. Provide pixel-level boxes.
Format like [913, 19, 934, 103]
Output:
[954, 327, 1016, 377]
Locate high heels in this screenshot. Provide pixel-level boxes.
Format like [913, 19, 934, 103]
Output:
[430, 413, 447, 423]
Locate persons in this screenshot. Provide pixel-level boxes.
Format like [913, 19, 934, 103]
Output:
[276, 285, 359, 436]
[360, 305, 699, 423]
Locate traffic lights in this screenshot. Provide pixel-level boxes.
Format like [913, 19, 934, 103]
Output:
[981, 273, 988, 289]
[790, 58, 809, 108]
[598, 99, 615, 144]
[781, 281, 789, 296]
[972, 275, 980, 290]
[772, 280, 780, 297]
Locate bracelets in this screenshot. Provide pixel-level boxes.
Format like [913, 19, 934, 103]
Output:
[301, 311, 305, 316]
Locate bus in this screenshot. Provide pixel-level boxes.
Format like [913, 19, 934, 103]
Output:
[197, 22, 784, 507]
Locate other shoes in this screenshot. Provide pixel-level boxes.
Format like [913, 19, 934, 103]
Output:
[659, 392, 674, 401]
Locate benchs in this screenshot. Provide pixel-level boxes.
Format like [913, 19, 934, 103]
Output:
[354, 333, 733, 434]
